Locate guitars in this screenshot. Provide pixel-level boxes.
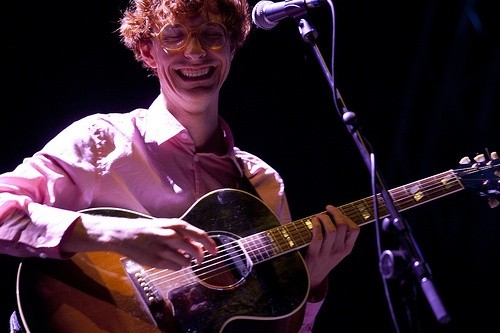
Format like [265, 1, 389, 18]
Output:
[16, 150, 500, 333]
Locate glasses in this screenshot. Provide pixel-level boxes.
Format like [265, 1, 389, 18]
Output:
[150, 21, 231, 50]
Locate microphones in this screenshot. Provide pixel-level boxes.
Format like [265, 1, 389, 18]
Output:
[251, 0, 327, 31]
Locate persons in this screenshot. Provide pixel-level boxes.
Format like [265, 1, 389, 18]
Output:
[0, 0, 360, 333]
